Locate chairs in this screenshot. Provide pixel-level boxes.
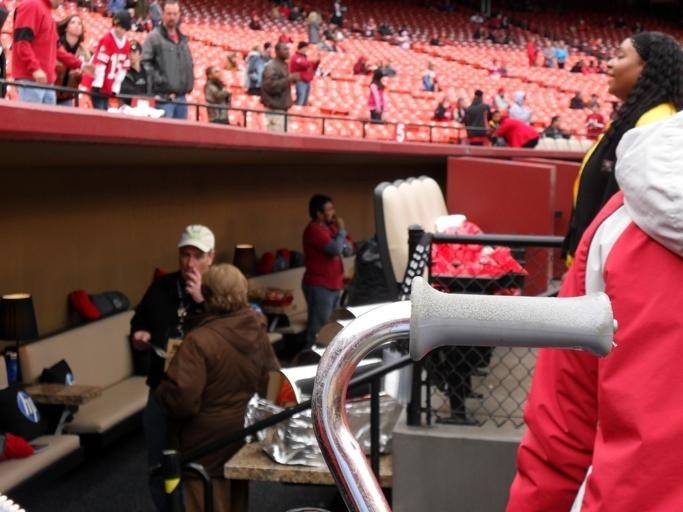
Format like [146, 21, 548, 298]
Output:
[0, 176, 527, 496]
[0, 0, 681, 152]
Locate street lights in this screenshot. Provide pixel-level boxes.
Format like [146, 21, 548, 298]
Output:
[258, 366, 298, 410]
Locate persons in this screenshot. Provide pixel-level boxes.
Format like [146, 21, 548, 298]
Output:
[301, 194, 358, 350]
[149, 262, 280, 512]
[130, 224, 216, 512]
[505, 110, 682, 512]
[559, 31, 683, 271]
[0, 0, 642, 147]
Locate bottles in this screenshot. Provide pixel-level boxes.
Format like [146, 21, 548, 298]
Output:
[178, 225, 215, 253]
[375, 69, 386, 76]
[129, 39, 142, 51]
